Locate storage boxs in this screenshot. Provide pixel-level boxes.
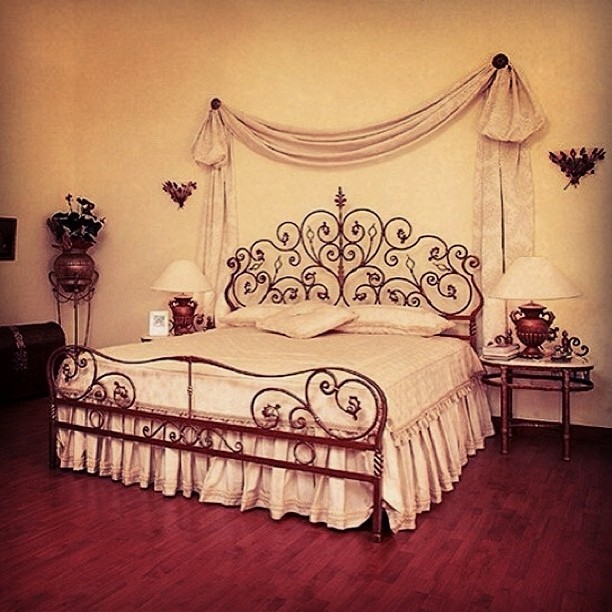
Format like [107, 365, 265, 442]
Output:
[0, 322, 64, 404]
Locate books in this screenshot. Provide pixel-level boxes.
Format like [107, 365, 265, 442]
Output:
[481, 344, 521, 360]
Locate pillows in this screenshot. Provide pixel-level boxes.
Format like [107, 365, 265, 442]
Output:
[332, 304, 456, 336]
[254, 300, 357, 338]
[218, 304, 290, 327]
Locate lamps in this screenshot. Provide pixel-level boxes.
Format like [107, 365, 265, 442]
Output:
[150, 260, 212, 336]
[488, 256, 581, 357]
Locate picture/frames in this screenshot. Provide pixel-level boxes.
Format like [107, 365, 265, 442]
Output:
[150, 309, 170, 336]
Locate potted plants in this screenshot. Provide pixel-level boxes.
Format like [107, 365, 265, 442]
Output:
[45, 192, 106, 290]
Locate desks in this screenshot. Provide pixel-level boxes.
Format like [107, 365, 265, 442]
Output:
[479, 350, 595, 460]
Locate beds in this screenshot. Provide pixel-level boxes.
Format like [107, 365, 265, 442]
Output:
[46, 185, 495, 543]
